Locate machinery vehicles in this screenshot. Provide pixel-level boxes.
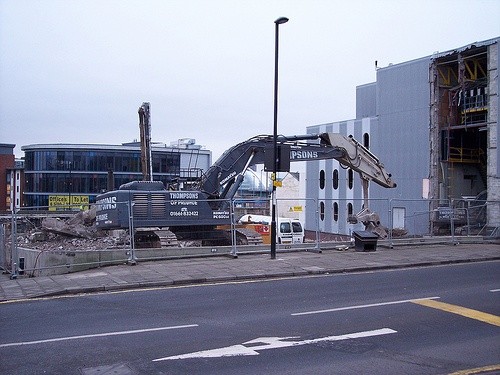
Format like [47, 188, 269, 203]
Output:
[95, 132, 396, 249]
[430, 190, 487, 235]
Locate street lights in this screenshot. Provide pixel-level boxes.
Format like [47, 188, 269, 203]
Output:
[270, 17, 290, 259]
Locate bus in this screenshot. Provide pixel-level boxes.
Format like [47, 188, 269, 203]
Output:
[241, 214, 305, 244]
